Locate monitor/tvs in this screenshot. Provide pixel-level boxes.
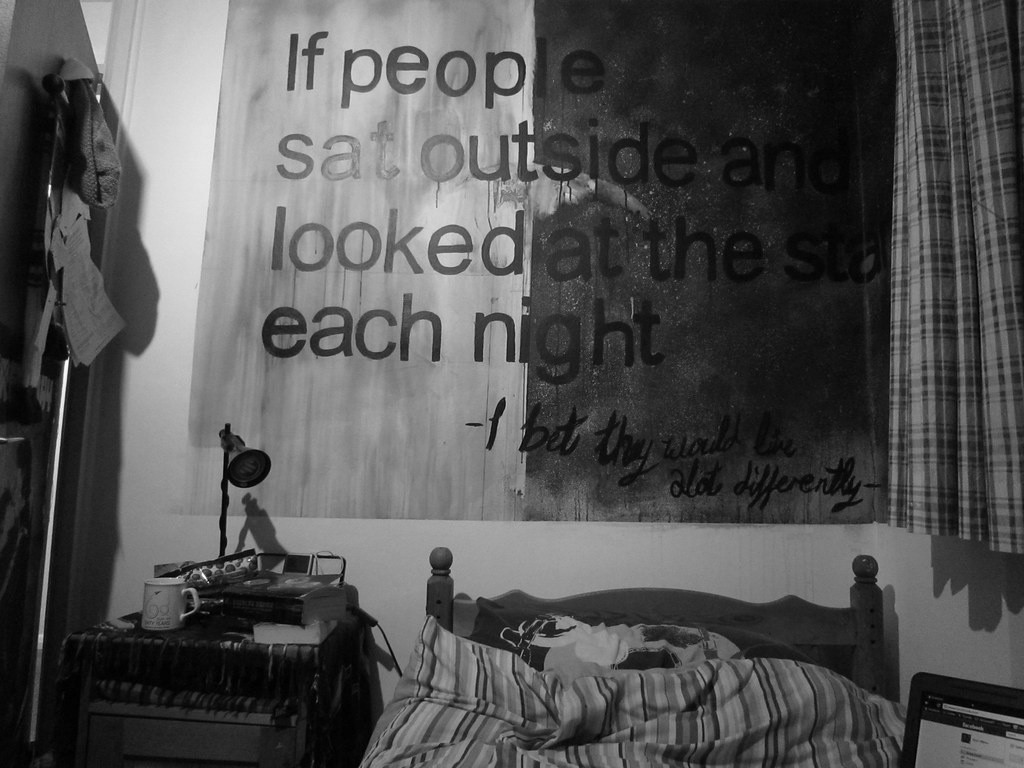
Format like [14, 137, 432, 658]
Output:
[899, 672, 1023, 768]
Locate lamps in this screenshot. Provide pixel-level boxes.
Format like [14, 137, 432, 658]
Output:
[218, 423, 271, 562]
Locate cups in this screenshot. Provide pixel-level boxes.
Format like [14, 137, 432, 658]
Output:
[139, 578, 199, 633]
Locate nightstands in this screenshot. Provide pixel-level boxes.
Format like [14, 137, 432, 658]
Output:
[54, 578, 353, 768]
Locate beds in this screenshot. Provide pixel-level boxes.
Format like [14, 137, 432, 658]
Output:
[360, 546, 910, 767]
